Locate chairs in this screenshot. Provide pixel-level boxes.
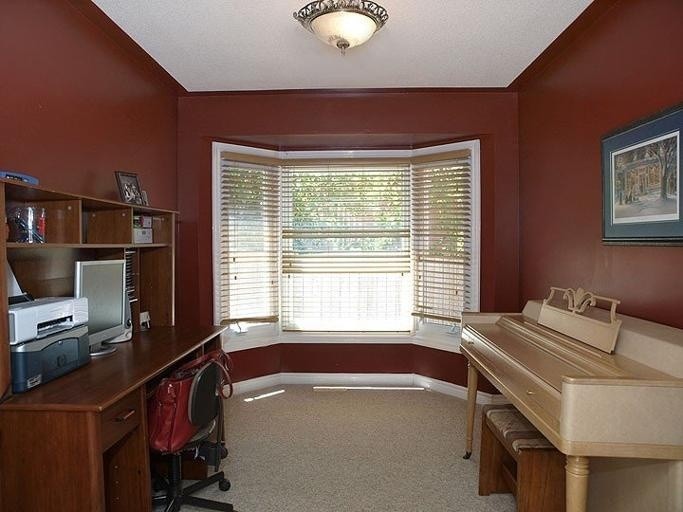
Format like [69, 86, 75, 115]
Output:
[152, 354, 235, 511]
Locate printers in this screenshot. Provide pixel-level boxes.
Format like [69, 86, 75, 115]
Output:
[6, 257, 89, 345]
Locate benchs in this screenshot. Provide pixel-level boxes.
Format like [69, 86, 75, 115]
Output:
[477, 403, 570, 511]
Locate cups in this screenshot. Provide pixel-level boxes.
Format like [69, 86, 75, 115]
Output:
[11, 205, 45, 243]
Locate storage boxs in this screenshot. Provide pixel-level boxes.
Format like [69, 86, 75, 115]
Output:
[133, 229, 154, 244]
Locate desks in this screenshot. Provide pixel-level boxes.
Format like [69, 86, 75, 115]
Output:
[1, 324, 229, 512]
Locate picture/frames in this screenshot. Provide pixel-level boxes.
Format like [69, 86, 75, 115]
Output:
[114, 170, 146, 205]
[599, 103, 683, 246]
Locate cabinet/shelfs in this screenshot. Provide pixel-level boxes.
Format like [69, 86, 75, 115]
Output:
[1, 175, 182, 327]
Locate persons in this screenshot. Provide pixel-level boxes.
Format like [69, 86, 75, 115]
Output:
[123, 183, 133, 203]
[130, 185, 141, 205]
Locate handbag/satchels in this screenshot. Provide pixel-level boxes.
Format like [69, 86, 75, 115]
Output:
[149, 348, 233, 452]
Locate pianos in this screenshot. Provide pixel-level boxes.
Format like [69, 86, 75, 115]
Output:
[460, 300, 683, 512]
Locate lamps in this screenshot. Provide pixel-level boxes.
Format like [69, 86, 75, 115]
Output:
[292, 1, 390, 54]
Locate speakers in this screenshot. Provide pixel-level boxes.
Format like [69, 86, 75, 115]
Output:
[106, 290, 133, 344]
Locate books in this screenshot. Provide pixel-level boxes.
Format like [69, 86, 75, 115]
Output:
[125, 250, 137, 303]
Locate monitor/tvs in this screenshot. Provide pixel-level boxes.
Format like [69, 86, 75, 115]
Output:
[75, 260, 125, 356]
